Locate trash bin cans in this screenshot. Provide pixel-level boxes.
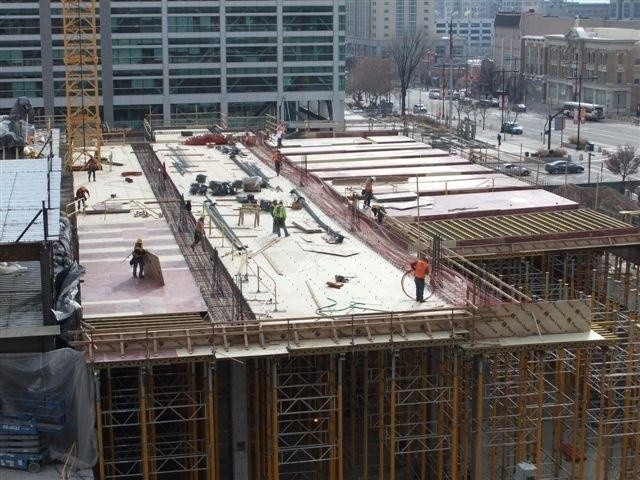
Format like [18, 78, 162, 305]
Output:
[589, 144, 594, 150]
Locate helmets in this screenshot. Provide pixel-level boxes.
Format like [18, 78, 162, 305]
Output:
[370, 176, 377, 182]
[137, 238, 143, 244]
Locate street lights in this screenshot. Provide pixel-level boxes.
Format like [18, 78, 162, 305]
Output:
[426, 48, 437, 90]
[441, 10, 461, 118]
[504, 58, 524, 98]
[434, 64, 457, 117]
[566, 76, 600, 150]
[490, 69, 521, 125]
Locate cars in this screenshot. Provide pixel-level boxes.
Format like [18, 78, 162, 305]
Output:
[351, 91, 393, 114]
[543, 160, 587, 175]
[411, 104, 428, 113]
[501, 121, 523, 135]
[426, 87, 528, 111]
[496, 163, 531, 176]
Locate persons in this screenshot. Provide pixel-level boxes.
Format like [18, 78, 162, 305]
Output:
[275, 202, 289, 237]
[372, 205, 386, 223]
[276, 123, 285, 146]
[191, 217, 206, 252]
[243, 131, 253, 147]
[132, 239, 146, 278]
[363, 176, 376, 208]
[273, 200, 277, 233]
[411, 256, 429, 302]
[85, 156, 98, 183]
[272, 150, 282, 177]
[497, 134, 501, 146]
[76, 187, 89, 209]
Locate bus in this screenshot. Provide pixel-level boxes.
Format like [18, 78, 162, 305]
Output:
[562, 101, 607, 122]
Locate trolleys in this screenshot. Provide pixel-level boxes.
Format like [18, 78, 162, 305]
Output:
[1, 419, 48, 474]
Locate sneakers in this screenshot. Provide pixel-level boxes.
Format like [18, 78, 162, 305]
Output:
[277, 233, 291, 238]
[132, 274, 144, 279]
[416, 297, 425, 303]
